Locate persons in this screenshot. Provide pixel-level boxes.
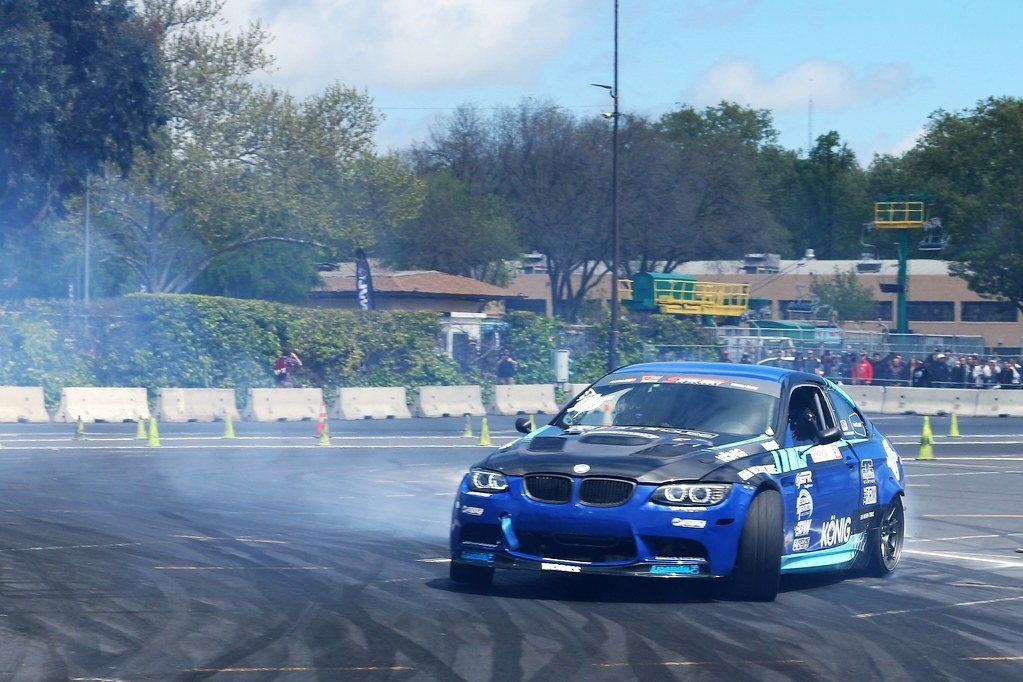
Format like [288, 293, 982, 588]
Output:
[718, 332, 1023, 390]
[496, 349, 518, 387]
[275, 350, 302, 389]
[613, 388, 669, 426]
[746, 401, 794, 449]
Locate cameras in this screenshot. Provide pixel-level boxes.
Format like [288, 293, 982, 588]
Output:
[502, 356, 508, 360]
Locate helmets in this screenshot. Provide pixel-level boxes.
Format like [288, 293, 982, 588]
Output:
[613, 389, 654, 425]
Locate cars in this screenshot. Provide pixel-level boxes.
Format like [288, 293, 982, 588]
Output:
[447, 360, 906, 604]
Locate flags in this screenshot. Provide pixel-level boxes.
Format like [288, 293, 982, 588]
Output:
[354, 248, 375, 310]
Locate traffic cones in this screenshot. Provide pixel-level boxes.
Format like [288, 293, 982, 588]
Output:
[915, 415, 938, 462]
[458, 414, 475, 438]
[475, 417, 495, 447]
[146, 417, 162, 448]
[72, 416, 89, 442]
[313, 402, 333, 438]
[133, 416, 149, 440]
[316, 420, 334, 447]
[947, 413, 965, 439]
[602, 404, 613, 427]
[221, 413, 238, 440]
